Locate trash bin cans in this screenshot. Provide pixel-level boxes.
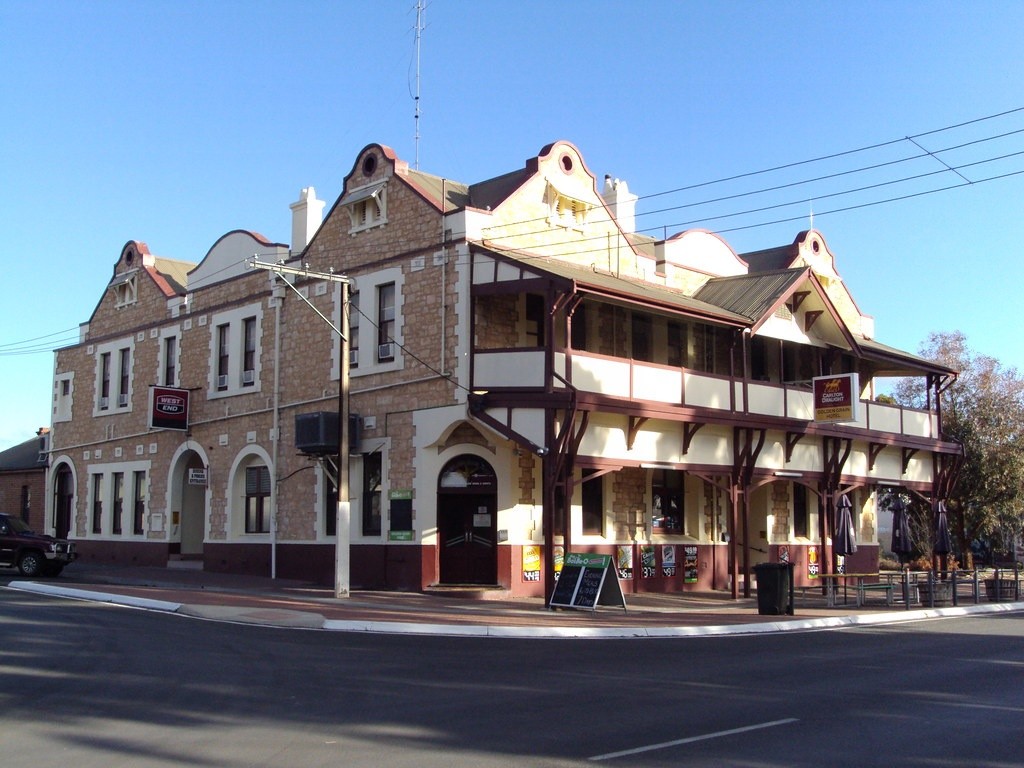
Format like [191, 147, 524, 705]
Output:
[751, 562, 797, 615]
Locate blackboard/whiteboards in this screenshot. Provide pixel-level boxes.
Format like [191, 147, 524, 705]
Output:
[547, 552, 611, 610]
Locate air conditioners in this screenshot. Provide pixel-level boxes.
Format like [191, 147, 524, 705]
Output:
[350, 350, 359, 364]
[218, 375, 227, 387]
[119, 394, 127, 404]
[242, 370, 254, 383]
[100, 397, 108, 407]
[378, 343, 394, 359]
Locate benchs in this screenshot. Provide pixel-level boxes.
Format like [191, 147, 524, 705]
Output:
[798, 580, 984, 607]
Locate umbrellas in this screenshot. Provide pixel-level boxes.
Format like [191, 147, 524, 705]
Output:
[834, 493, 858, 602]
[932, 499, 953, 580]
[891, 497, 913, 581]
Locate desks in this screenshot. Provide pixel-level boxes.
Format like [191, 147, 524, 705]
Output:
[808, 570, 987, 608]
[785, 379, 812, 389]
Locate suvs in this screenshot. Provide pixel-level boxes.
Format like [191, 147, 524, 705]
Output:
[0, 514, 80, 579]
